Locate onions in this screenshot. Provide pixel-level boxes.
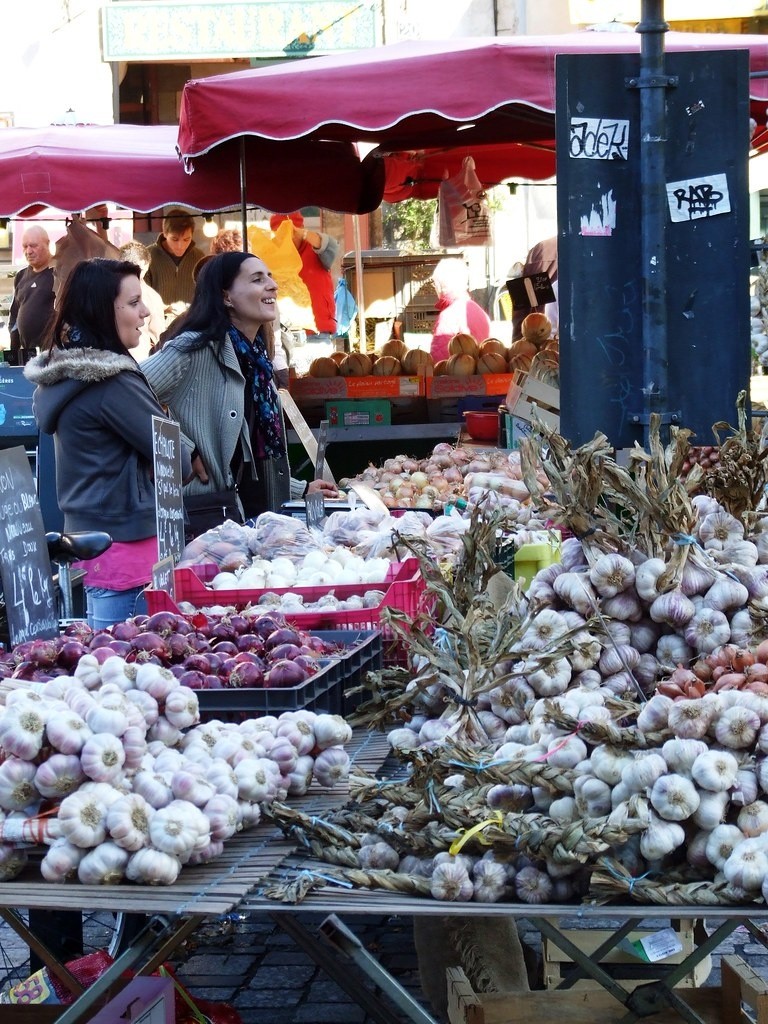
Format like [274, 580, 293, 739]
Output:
[343, 440, 547, 508]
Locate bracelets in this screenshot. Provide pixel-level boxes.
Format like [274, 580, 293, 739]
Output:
[303, 230, 307, 240]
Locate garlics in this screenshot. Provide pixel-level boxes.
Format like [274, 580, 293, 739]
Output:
[0, 495, 768, 902]
[750, 296, 768, 366]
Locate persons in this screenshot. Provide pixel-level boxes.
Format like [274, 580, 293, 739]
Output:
[116, 240, 167, 366]
[23, 259, 193, 631]
[144, 209, 204, 307]
[9, 225, 59, 367]
[431, 258, 491, 365]
[210, 229, 294, 389]
[138, 252, 338, 541]
[269, 209, 337, 374]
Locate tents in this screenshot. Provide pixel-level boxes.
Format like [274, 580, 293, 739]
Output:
[0, 29, 768, 256]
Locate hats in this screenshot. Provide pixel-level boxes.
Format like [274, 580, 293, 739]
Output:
[269, 209, 305, 231]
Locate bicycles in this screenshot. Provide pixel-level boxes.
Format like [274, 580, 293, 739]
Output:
[0, 527, 133, 991]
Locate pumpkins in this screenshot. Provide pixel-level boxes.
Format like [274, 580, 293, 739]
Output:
[310, 311, 551, 374]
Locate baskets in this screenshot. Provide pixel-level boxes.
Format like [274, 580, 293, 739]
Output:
[173, 556, 439, 606]
[145, 568, 428, 667]
[163, 629, 384, 715]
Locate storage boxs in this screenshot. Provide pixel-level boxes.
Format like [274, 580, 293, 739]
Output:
[456, 392, 506, 422]
[0, 364, 39, 436]
[288, 362, 560, 449]
[145, 555, 438, 733]
[0, 975, 176, 1024]
